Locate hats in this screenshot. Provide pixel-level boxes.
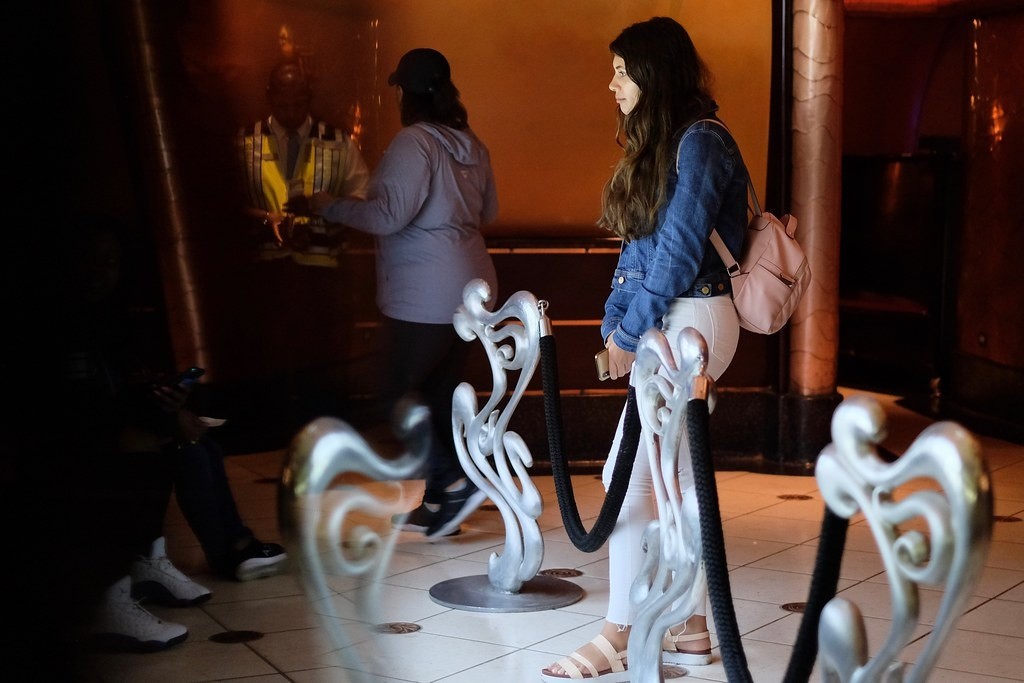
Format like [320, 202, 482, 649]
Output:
[388, 48, 451, 94]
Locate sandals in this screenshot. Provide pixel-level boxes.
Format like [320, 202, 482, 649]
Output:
[662, 628, 712, 666]
[540, 632, 630, 683]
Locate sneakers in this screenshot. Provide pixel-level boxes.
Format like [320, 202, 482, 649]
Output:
[425, 478, 488, 543]
[390, 502, 461, 536]
[130, 536, 212, 609]
[88, 575, 188, 654]
[234, 542, 288, 581]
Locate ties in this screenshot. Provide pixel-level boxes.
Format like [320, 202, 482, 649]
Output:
[287, 128, 300, 179]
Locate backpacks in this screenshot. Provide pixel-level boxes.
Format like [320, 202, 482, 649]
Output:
[676, 119, 813, 335]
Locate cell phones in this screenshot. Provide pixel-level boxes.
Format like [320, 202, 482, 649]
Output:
[595, 349, 610, 381]
[174, 366, 205, 383]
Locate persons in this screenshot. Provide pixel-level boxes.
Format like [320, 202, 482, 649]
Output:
[44, 217, 287, 652]
[542, 17, 751, 683]
[283, 47, 500, 540]
